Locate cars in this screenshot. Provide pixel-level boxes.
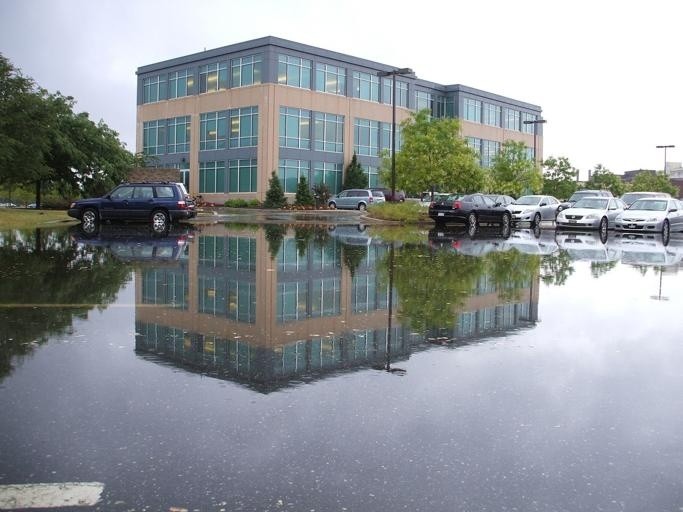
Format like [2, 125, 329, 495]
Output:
[428, 225, 683, 268]
[366, 186, 404, 202]
[412, 190, 682, 234]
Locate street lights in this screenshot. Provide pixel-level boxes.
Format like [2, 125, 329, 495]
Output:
[523, 119, 546, 169]
[375, 67, 412, 202]
[655, 145, 674, 175]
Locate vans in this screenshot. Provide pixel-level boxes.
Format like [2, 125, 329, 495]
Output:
[326, 188, 385, 211]
[327, 224, 373, 247]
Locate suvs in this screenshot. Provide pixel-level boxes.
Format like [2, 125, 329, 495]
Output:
[67, 179, 197, 223]
[68, 221, 195, 264]
